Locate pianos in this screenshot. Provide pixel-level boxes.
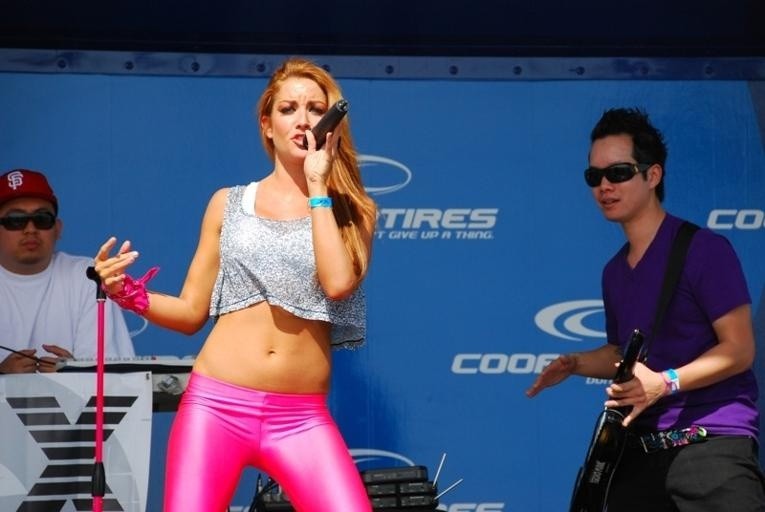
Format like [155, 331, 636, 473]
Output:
[54, 356, 200, 412]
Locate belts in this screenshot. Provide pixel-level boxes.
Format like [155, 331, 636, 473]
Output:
[628, 427, 708, 453]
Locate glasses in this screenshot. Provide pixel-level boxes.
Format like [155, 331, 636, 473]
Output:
[584, 162, 651, 187]
[0, 208, 54, 230]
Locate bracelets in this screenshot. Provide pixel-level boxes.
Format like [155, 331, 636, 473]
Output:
[658, 365, 683, 396]
[99, 266, 161, 317]
[302, 197, 335, 210]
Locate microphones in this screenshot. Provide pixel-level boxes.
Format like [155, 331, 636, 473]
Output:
[304, 99, 350, 151]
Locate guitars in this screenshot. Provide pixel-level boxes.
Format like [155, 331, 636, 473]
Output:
[569, 327, 649, 509]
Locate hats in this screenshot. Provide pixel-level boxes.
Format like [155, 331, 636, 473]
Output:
[0, 169, 57, 206]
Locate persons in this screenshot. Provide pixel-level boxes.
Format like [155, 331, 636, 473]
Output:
[92, 58, 378, 511]
[527, 105, 764, 509]
[0, 168, 140, 375]
